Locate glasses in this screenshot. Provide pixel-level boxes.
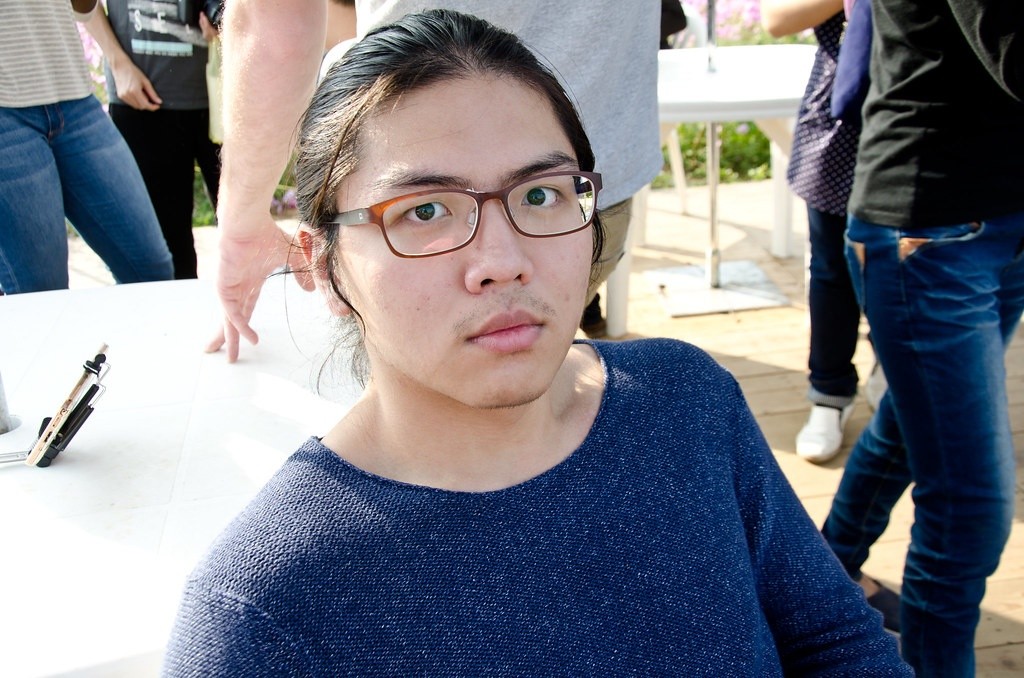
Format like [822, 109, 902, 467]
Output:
[320, 170, 602, 258]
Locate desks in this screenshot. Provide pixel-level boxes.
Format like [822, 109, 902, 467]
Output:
[656, 42, 818, 318]
[1, 276, 370, 678]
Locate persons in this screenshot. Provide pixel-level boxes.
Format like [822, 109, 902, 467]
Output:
[0, 0, 663, 364]
[757, 2, 1024, 677]
[158, 9, 919, 677]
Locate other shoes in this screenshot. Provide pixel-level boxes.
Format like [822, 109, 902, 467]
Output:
[857, 574, 903, 635]
[797, 403, 854, 463]
[580, 315, 607, 339]
